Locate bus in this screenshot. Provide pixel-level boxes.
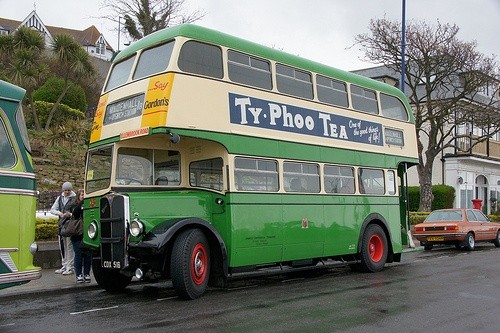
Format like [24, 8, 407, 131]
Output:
[0, 79, 42, 290]
[82, 23, 424, 300]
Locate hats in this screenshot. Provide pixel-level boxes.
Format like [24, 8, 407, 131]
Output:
[62, 182, 72, 191]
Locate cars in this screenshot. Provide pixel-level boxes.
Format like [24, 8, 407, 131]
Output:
[413, 208, 500, 251]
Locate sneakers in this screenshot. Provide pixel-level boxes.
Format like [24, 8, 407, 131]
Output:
[76, 273, 84, 283]
[55, 267, 65, 273]
[62, 270, 72, 274]
[84, 274, 91, 282]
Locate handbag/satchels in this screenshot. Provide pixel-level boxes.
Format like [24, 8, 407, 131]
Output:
[61, 208, 83, 236]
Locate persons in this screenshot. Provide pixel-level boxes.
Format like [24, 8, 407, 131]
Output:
[50, 181, 77, 275]
[67, 189, 92, 284]
